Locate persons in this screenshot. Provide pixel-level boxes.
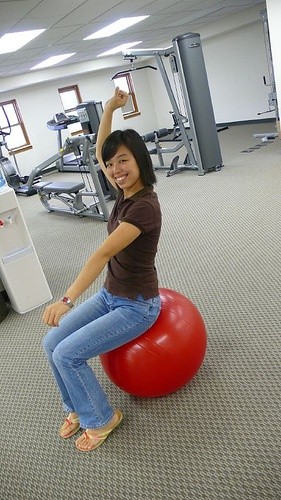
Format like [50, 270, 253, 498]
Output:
[41, 87, 162, 451]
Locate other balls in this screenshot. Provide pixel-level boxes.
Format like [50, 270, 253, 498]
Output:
[99, 288, 207, 398]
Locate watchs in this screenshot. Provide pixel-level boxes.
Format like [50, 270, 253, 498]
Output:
[61, 296, 74, 308]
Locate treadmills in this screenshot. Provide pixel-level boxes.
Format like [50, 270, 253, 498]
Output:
[46, 112, 91, 173]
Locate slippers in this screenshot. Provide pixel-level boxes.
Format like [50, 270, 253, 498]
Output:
[59, 412, 81, 438]
[75, 410, 123, 451]
